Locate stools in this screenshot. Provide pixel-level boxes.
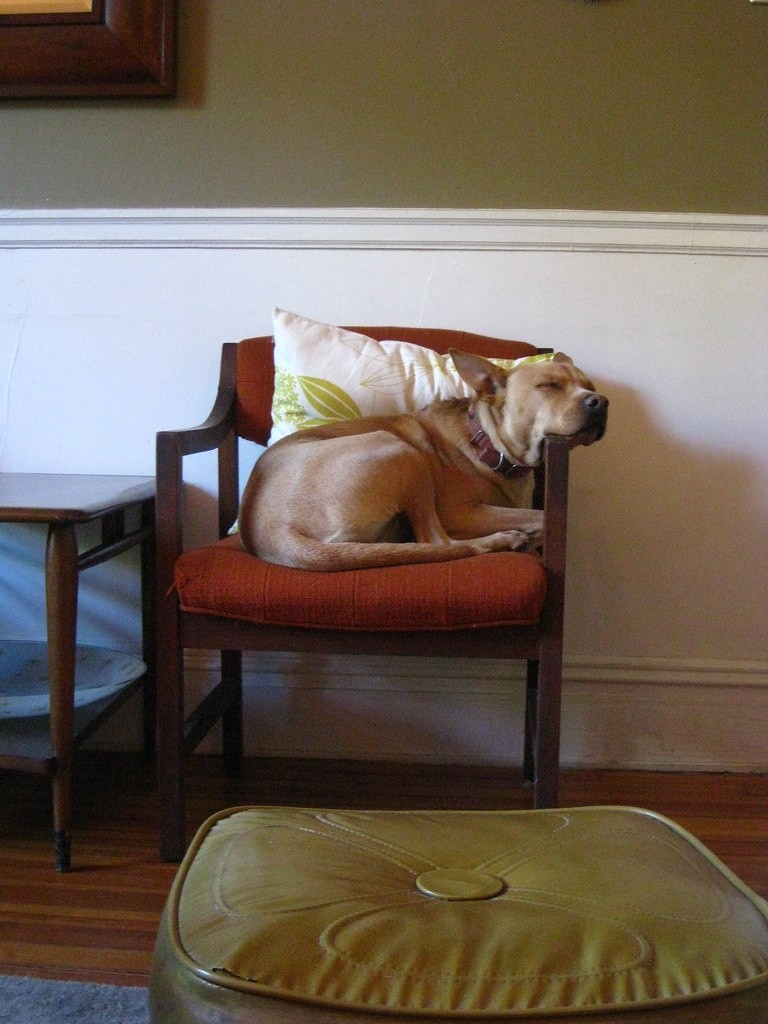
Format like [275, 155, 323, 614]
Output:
[148, 798, 768, 1024]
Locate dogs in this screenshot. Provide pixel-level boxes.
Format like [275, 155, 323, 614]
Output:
[237, 348, 611, 572]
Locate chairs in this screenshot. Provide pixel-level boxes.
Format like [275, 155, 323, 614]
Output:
[149, 330, 566, 863]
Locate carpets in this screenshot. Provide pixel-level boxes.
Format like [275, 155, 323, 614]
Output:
[0, 972, 152, 1024]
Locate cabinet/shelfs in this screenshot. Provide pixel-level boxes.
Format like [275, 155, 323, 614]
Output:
[0, 471, 155, 873]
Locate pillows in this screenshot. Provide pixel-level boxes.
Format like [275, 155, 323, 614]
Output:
[227, 306, 539, 546]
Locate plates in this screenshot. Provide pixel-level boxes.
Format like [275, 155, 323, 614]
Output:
[0, 640, 148, 720]
